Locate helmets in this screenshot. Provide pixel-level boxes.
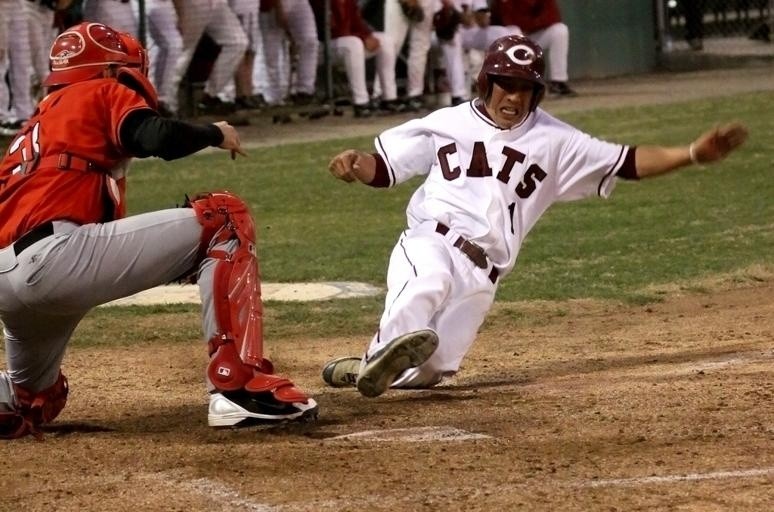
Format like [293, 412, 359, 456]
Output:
[42, 20, 149, 91]
[477, 35, 546, 112]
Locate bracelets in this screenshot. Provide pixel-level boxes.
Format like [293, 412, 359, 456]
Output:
[689, 140, 709, 171]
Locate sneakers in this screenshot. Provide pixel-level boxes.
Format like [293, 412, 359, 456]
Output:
[544, 80, 577, 100]
[0, 116, 31, 137]
[356, 329, 441, 399]
[207, 382, 319, 428]
[323, 356, 361, 389]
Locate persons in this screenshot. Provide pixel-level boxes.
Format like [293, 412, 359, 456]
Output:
[0, 20, 319, 440]
[320, 34, 752, 398]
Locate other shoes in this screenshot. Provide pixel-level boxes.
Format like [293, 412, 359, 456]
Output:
[351, 95, 426, 118]
[292, 94, 329, 120]
[272, 104, 292, 125]
[160, 94, 269, 127]
[450, 96, 464, 108]
